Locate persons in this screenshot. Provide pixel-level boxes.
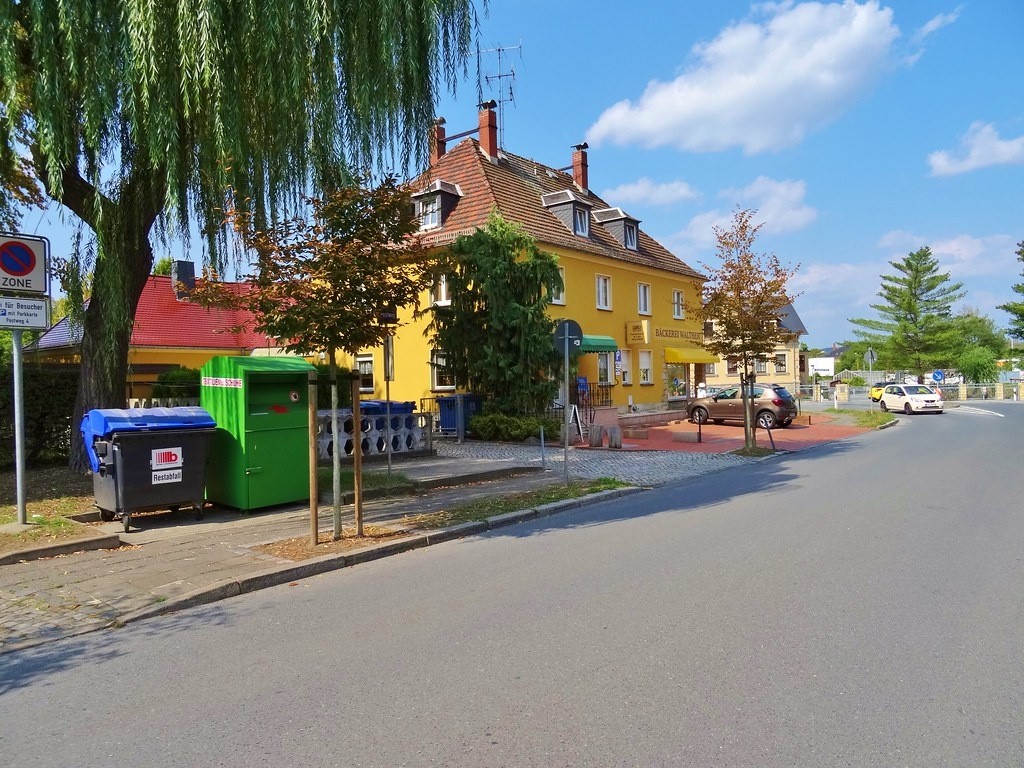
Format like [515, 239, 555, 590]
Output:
[697, 382, 706, 398]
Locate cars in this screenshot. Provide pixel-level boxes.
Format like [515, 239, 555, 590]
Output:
[881, 384, 946, 416]
[686, 382, 797, 429]
[868, 381, 899, 405]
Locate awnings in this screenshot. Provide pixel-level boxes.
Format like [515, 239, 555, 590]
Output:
[579, 335, 618, 354]
[664, 347, 720, 364]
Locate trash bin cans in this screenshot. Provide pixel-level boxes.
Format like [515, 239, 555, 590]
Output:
[79, 404, 219, 535]
[435, 393, 484, 437]
[359, 399, 418, 449]
